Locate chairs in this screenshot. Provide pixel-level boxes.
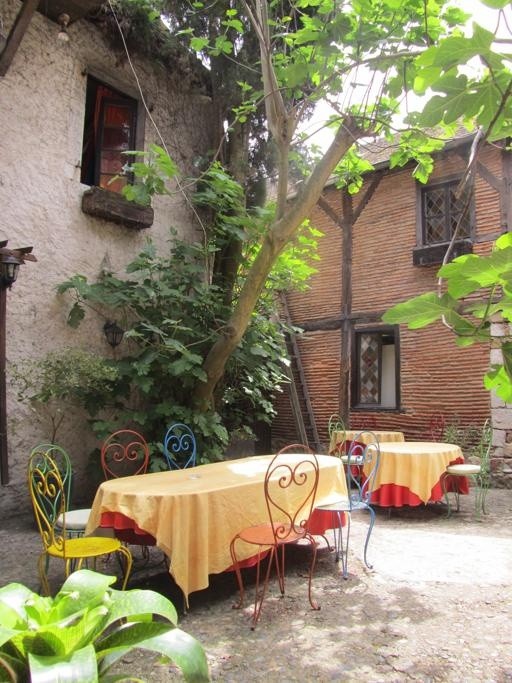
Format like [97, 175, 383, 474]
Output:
[228, 442, 319, 630]
[100, 428, 170, 584]
[26, 444, 133, 598]
[164, 424, 197, 471]
[440, 418, 494, 517]
[313, 431, 380, 579]
[328, 413, 365, 501]
[431, 410, 446, 443]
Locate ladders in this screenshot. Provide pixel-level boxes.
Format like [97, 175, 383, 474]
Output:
[267, 286, 322, 453]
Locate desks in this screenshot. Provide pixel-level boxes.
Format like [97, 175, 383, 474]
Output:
[82, 454, 349, 616]
[361, 441, 470, 520]
[327, 429, 405, 486]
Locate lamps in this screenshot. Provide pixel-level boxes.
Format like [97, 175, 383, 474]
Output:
[103, 321, 124, 348]
[0, 255, 20, 290]
